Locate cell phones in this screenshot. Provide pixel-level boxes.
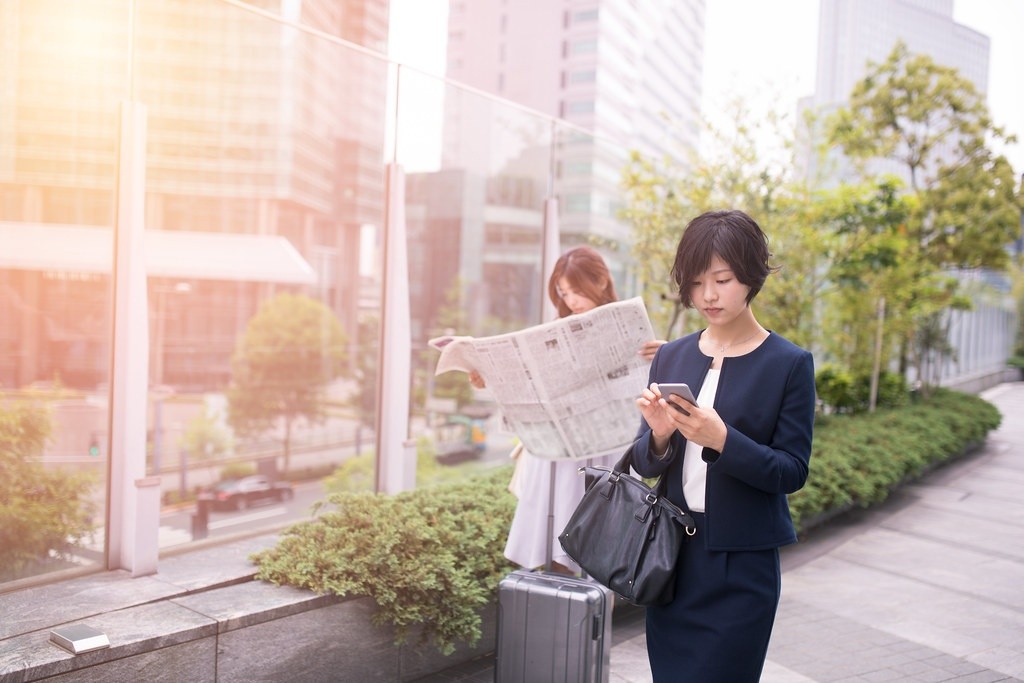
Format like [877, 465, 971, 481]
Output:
[658, 383, 699, 417]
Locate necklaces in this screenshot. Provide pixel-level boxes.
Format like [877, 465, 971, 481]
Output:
[705, 328, 762, 351]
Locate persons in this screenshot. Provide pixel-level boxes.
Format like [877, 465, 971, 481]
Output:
[630, 209, 815, 683]
[470, 246, 668, 577]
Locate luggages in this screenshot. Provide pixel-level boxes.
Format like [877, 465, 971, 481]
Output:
[493, 457, 614, 683]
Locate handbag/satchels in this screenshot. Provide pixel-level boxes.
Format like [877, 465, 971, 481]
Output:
[558, 437, 697, 607]
